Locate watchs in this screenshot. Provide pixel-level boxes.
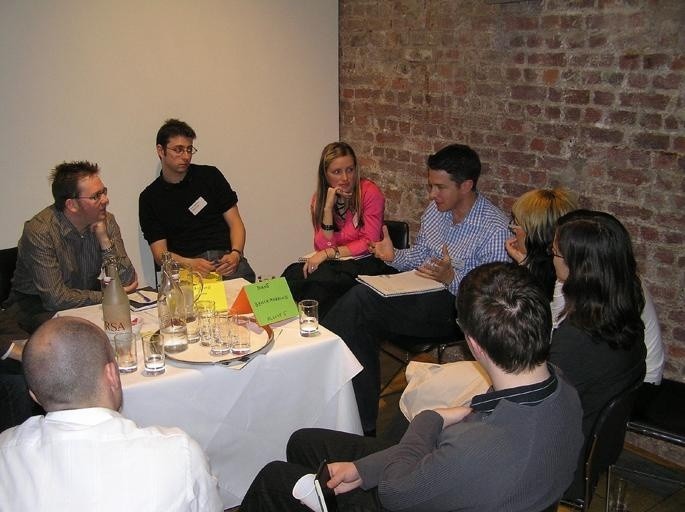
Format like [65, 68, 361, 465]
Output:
[329, 245, 340, 259]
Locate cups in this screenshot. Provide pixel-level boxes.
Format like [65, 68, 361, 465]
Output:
[293, 473, 323, 512]
[114, 332, 138, 375]
[175, 261, 204, 324]
[141, 333, 166, 373]
[129, 313, 143, 339]
[297, 299, 320, 338]
[186, 298, 251, 355]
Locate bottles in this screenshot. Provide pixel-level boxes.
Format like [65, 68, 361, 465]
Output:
[100, 248, 133, 347]
[156, 251, 189, 354]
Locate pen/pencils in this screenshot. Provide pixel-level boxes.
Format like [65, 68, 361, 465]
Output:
[136, 290, 151, 302]
[214, 261, 230, 268]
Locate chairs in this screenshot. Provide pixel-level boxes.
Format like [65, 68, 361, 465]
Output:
[561, 380, 641, 511]
[377, 222, 409, 251]
[1, 241, 17, 308]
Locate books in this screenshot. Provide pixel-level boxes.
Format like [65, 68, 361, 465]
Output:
[126, 285, 159, 311]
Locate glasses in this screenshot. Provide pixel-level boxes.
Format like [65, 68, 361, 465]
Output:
[546, 242, 565, 259]
[167, 146, 197, 154]
[508, 218, 521, 230]
[72, 187, 107, 200]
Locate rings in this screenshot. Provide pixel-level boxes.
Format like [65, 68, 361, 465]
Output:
[311, 265, 314, 271]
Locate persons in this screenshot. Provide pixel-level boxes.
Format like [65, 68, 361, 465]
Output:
[238, 262, 585, 511]
[0, 332, 45, 433]
[0, 317, 225, 511]
[505, 188, 580, 305]
[549, 208, 648, 487]
[137, 118, 257, 284]
[322, 144, 516, 438]
[281, 142, 390, 323]
[5, 160, 138, 332]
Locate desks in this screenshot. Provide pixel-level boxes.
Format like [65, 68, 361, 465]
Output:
[45, 272, 366, 511]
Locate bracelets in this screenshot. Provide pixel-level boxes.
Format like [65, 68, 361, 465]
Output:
[230, 248, 242, 255]
[323, 249, 329, 260]
[320, 220, 335, 231]
[99, 245, 115, 252]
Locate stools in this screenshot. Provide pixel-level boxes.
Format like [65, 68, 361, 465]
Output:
[373, 333, 471, 396]
[604, 377, 685, 510]
